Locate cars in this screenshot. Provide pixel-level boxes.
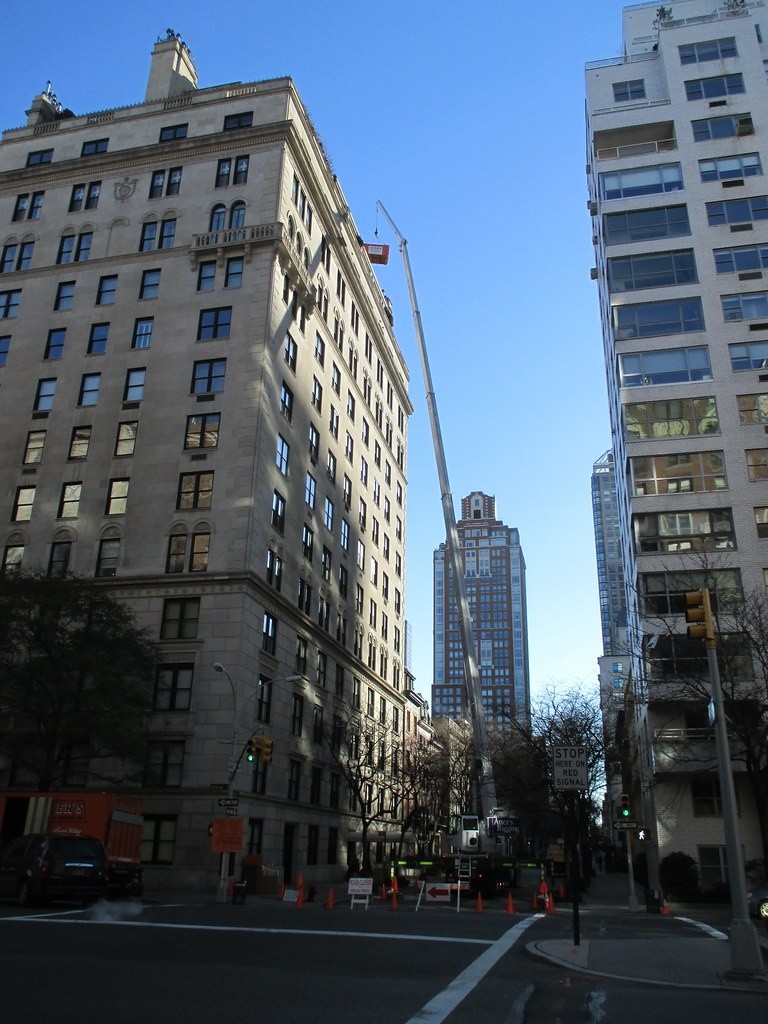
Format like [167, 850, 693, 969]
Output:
[748, 884, 768, 919]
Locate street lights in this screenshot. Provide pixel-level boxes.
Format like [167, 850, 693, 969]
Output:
[213, 661, 302, 903]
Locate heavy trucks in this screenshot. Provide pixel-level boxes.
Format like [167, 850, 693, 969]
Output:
[0, 791, 146, 896]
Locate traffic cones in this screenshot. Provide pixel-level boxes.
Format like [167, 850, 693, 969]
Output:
[662, 897, 671, 914]
[529, 890, 539, 908]
[504, 891, 516, 914]
[475, 891, 485, 912]
[379, 884, 387, 900]
[295, 887, 305, 906]
[559, 884, 565, 897]
[544, 892, 556, 915]
[324, 888, 336, 909]
[389, 892, 400, 911]
[276, 885, 285, 900]
[391, 876, 402, 896]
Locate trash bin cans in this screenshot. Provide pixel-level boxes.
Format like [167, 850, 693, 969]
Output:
[233, 880, 247, 906]
[645, 890, 660, 914]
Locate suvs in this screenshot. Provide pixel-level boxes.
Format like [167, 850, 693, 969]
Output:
[0, 832, 111, 906]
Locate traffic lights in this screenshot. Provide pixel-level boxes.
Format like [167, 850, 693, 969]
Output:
[684, 589, 709, 639]
[634, 828, 651, 840]
[246, 737, 256, 762]
[620, 794, 630, 817]
[261, 737, 273, 764]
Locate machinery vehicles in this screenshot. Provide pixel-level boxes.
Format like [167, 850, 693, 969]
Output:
[374, 199, 548, 898]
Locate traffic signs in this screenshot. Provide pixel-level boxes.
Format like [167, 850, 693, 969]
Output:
[612, 821, 639, 829]
[225, 809, 237, 815]
[217, 799, 238, 806]
